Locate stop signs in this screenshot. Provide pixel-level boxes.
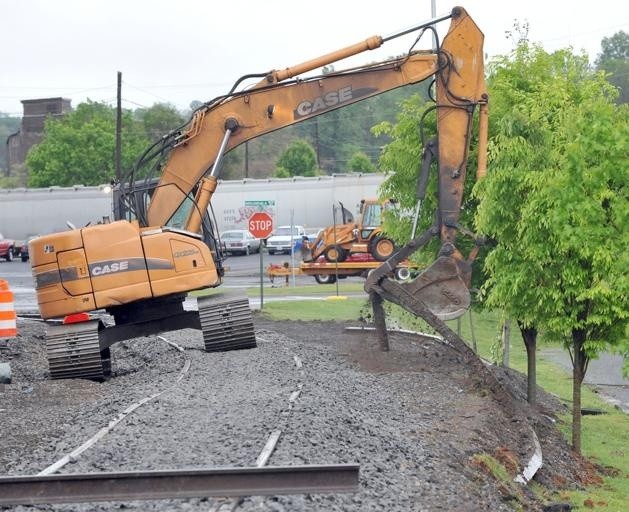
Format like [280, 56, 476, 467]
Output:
[246, 210, 274, 241]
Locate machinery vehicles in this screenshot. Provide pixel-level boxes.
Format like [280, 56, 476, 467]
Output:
[19, 1, 501, 389]
[284, 190, 406, 264]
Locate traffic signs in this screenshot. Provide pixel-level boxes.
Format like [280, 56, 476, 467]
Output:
[244, 200, 275, 207]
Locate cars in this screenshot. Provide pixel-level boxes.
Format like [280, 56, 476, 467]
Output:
[0, 232, 44, 265]
[263, 221, 305, 256]
[303, 226, 325, 243]
[217, 228, 262, 256]
